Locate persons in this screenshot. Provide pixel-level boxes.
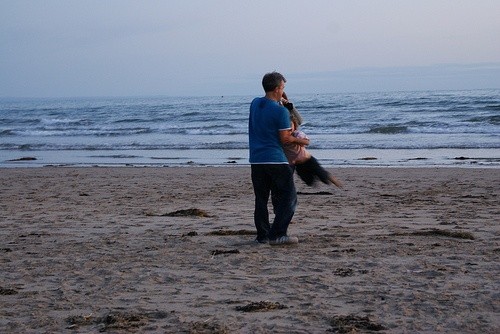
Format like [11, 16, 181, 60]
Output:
[279, 113, 343, 191]
[248, 69, 310, 246]
[271, 91, 304, 224]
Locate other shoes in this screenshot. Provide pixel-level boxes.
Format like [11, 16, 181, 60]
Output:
[271, 236, 298, 247]
[329, 175, 341, 189]
